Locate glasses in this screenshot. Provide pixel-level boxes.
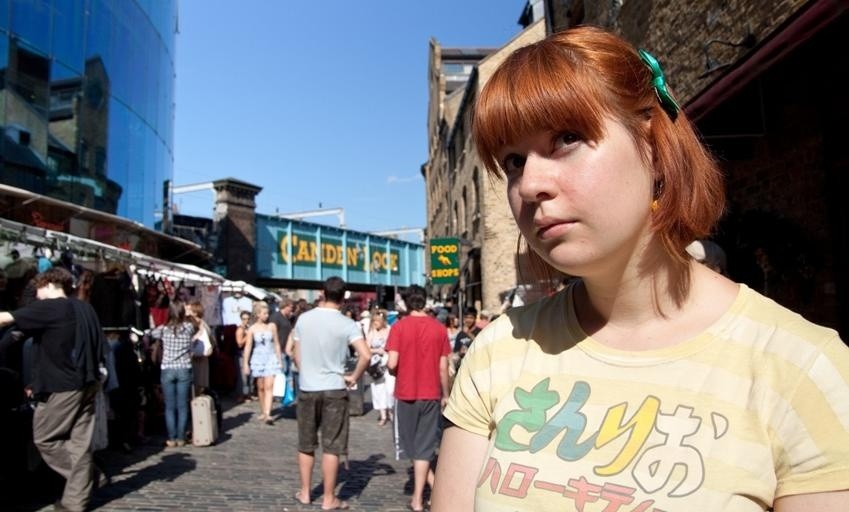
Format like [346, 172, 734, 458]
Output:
[260, 334, 265, 345]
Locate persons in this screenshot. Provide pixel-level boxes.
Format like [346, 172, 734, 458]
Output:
[291, 275, 372, 512]
[683, 240, 730, 276]
[384, 284, 452, 511]
[0, 268, 109, 511]
[1, 297, 487, 512]
[432, 23, 849, 510]
[751, 244, 778, 300]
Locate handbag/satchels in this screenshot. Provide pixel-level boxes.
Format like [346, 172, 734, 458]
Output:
[279, 380, 295, 409]
[272, 370, 286, 397]
[366, 353, 386, 379]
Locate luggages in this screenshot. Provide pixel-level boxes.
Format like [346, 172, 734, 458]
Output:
[188, 383, 219, 447]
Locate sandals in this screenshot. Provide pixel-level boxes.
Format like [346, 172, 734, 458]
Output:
[377, 417, 387, 426]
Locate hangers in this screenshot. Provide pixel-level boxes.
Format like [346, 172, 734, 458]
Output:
[16, 240, 202, 304]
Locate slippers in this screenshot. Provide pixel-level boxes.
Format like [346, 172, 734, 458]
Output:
[406, 501, 424, 512]
[321, 498, 349, 510]
[293, 491, 311, 505]
